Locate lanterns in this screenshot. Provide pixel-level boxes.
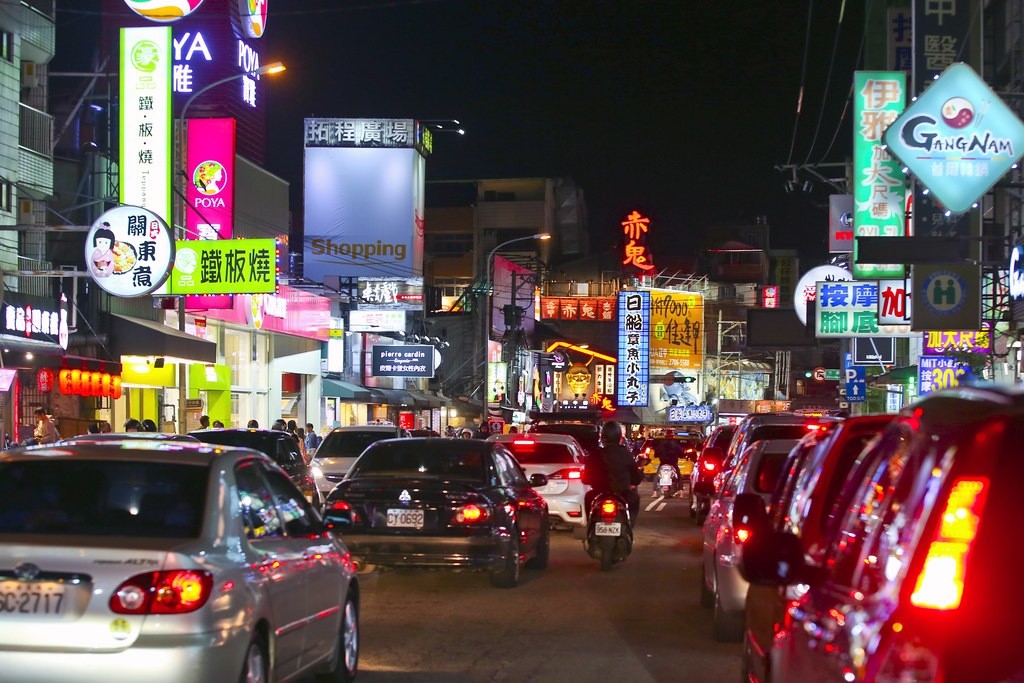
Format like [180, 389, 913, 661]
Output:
[38, 368, 122, 399]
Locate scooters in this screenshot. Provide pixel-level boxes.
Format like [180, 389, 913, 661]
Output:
[576, 455, 651, 572]
[657, 463, 681, 499]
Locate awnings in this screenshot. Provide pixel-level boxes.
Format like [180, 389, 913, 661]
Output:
[324, 379, 451, 409]
[706, 359, 773, 373]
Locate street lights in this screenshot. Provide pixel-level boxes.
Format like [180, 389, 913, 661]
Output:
[483, 231, 551, 422]
[178, 62, 287, 439]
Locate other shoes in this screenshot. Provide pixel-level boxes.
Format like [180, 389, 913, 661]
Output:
[680, 493, 684, 498]
[651, 493, 658, 498]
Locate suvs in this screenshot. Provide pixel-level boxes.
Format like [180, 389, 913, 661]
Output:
[527, 420, 604, 455]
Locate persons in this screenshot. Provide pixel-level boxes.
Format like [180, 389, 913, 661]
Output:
[33, 408, 56, 444]
[194, 416, 223, 430]
[88, 419, 157, 434]
[651, 431, 686, 499]
[584, 422, 641, 531]
[247, 419, 322, 463]
[442, 421, 517, 440]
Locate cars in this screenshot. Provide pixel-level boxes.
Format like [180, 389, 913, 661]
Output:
[682, 381, 1024, 683]
[320, 437, 550, 589]
[484, 433, 594, 532]
[309, 425, 412, 498]
[186, 428, 321, 513]
[408, 426, 441, 438]
[0, 440, 363, 683]
[629, 424, 704, 482]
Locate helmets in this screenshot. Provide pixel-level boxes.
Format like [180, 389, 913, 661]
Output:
[509, 426, 517, 433]
[141, 419, 157, 432]
[461, 428, 473, 439]
[479, 421, 489, 432]
[665, 430, 674, 439]
[124, 419, 142, 433]
[601, 421, 622, 445]
